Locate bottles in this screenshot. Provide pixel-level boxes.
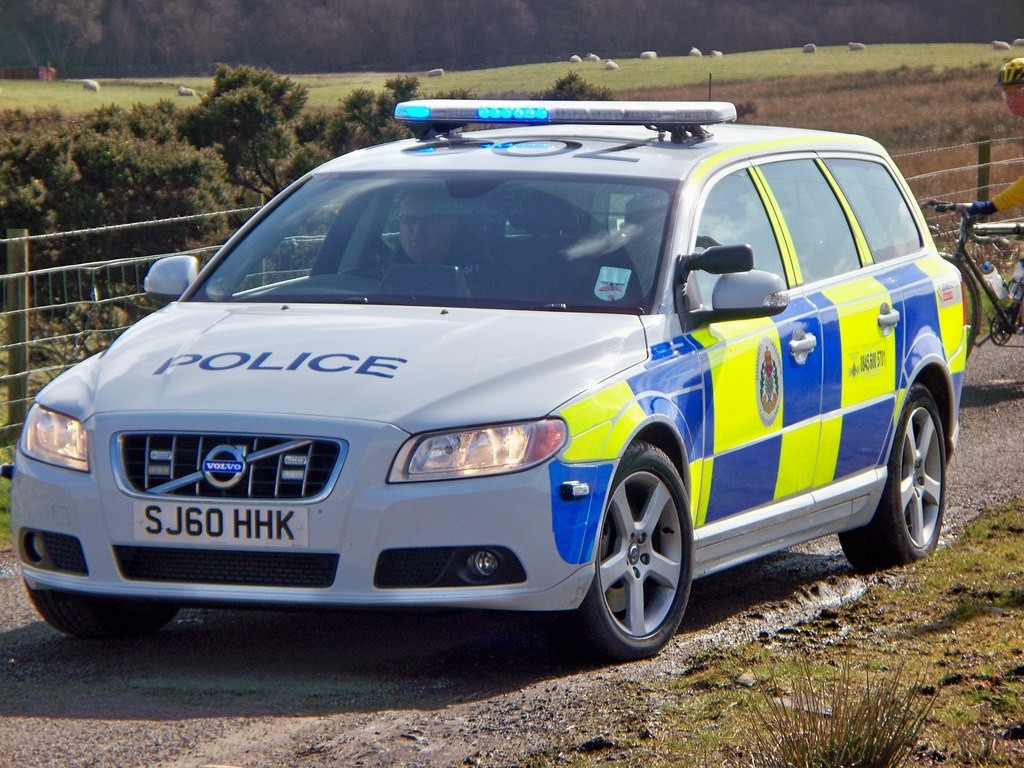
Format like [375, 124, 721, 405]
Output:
[1007, 261, 1024, 300]
[982, 263, 1008, 300]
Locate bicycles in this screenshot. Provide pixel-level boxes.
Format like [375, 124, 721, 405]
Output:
[918, 197, 1024, 361]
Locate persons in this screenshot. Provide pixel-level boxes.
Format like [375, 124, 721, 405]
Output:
[352, 184, 502, 297]
[967, 58, 1024, 333]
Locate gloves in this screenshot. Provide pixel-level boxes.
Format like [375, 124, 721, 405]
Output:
[965, 201, 997, 215]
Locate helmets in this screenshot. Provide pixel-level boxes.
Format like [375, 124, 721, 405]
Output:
[995, 58, 1024, 86]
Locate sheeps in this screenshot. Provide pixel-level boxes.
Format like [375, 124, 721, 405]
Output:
[640, 51, 656, 59]
[992, 40, 1009, 50]
[1013, 38, 1024, 46]
[570, 55, 581, 64]
[586, 53, 599, 62]
[604, 59, 618, 69]
[428, 68, 443, 77]
[178, 86, 195, 96]
[688, 47, 702, 56]
[711, 50, 722, 56]
[848, 42, 865, 50]
[802, 43, 815, 53]
[82, 79, 99, 93]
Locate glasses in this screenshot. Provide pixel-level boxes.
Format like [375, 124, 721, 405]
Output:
[396, 212, 448, 225]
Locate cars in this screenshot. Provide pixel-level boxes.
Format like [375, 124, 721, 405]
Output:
[13, 98, 971, 660]
